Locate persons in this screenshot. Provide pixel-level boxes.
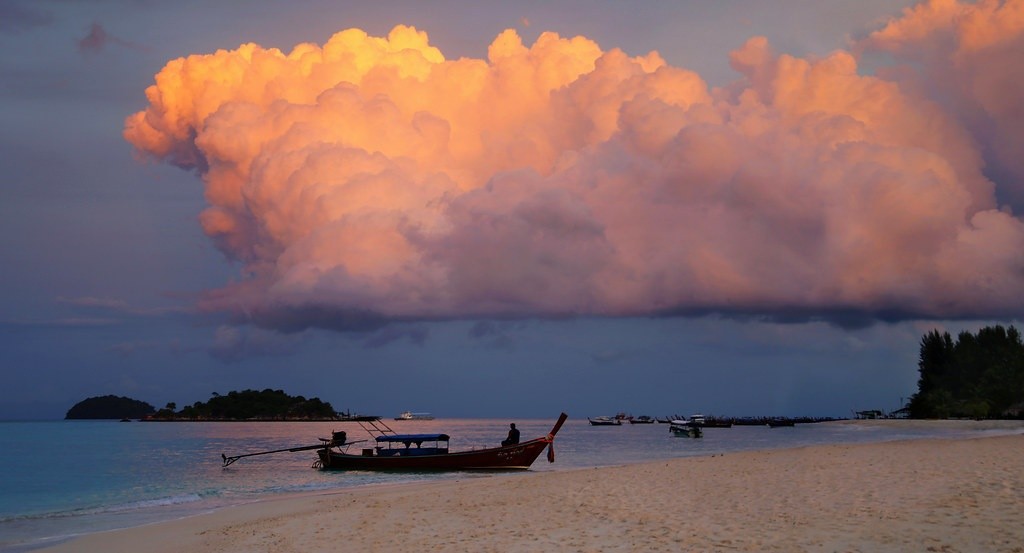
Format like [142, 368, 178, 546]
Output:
[501, 423, 520, 447]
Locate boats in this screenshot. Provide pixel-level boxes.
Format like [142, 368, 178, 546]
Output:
[586, 410, 795, 429]
[394, 412, 434, 421]
[670, 420, 703, 438]
[315, 410, 569, 472]
